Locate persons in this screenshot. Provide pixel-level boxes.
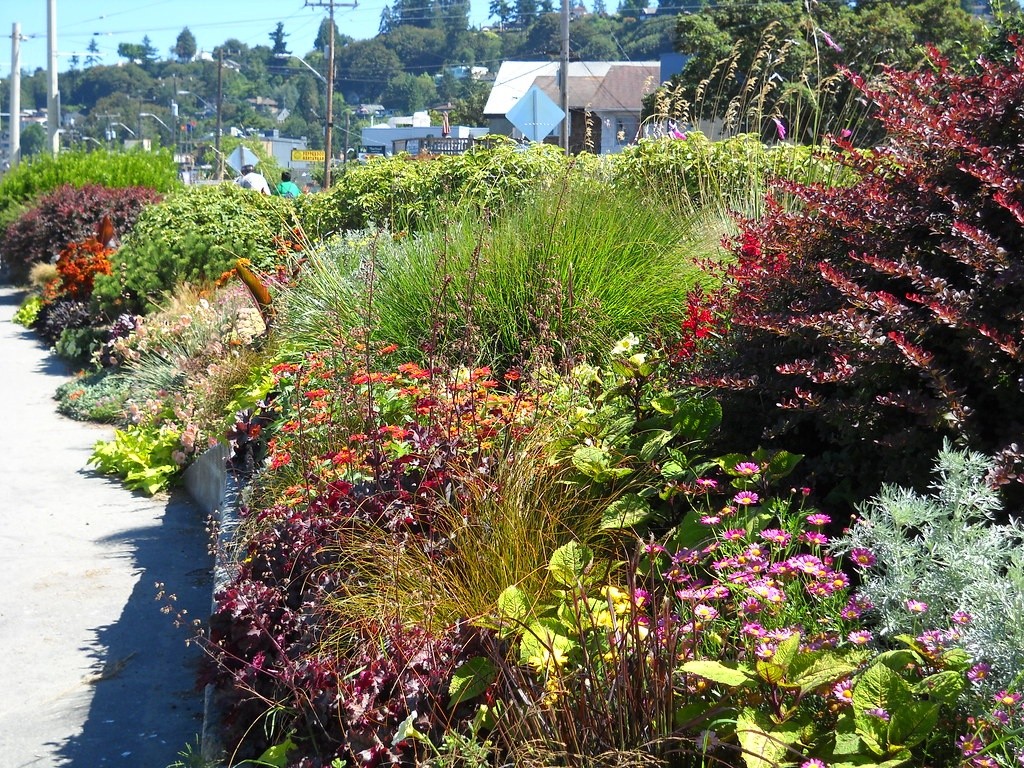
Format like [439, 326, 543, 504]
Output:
[273, 173, 302, 198]
[238, 165, 271, 195]
[442, 110, 450, 137]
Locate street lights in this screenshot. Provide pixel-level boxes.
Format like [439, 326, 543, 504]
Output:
[273, 52, 334, 189]
[139, 111, 176, 147]
[176, 90, 221, 180]
